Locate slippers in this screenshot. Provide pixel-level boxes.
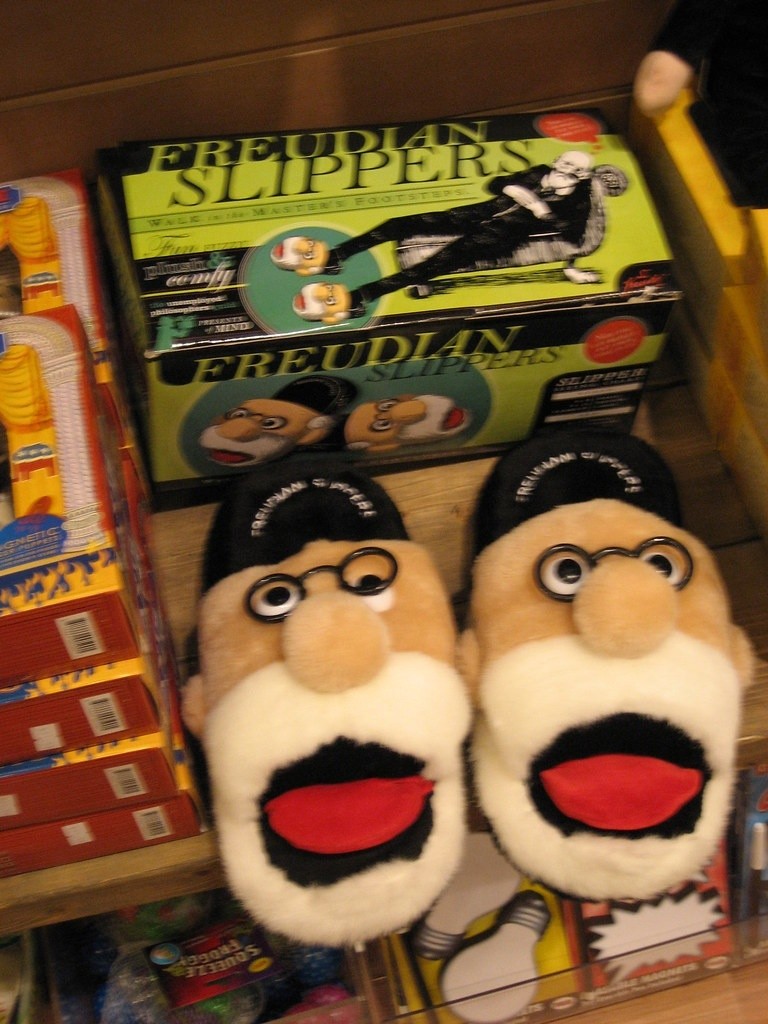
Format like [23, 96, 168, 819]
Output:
[182, 457, 476, 949]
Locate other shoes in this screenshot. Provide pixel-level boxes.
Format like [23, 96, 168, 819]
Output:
[458, 418, 754, 907]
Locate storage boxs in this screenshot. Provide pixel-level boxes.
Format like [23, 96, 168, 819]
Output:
[0, 107, 692, 879]
